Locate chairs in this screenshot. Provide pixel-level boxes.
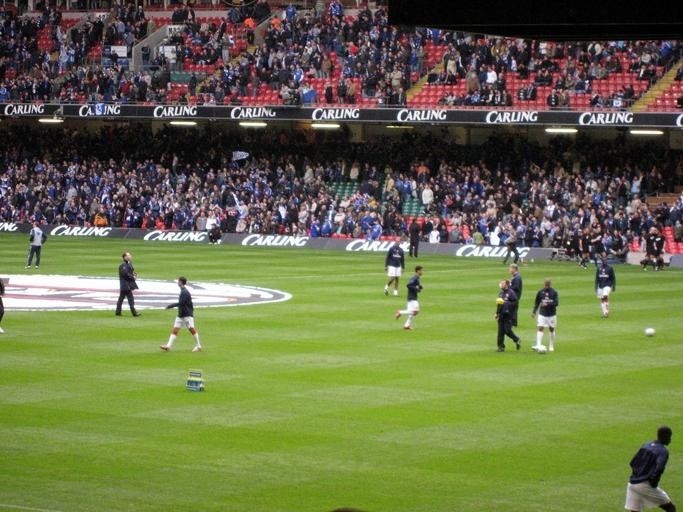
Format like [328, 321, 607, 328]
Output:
[280, 140, 471, 245]
[112, 203, 179, 229]
[613, 177, 682, 255]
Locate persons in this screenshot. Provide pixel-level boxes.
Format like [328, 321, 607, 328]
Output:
[0, 1, 683, 107]
[529, 278, 560, 356]
[622, 425, 677, 512]
[508, 262, 522, 329]
[113, 247, 142, 318]
[0, 124, 683, 268]
[158, 276, 202, 352]
[493, 279, 521, 352]
[392, 264, 424, 337]
[593, 251, 615, 319]
[0, 277, 10, 334]
[380, 229, 406, 297]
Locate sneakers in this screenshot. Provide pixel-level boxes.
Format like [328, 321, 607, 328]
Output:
[395, 309, 402, 321]
[159, 344, 171, 354]
[191, 345, 203, 354]
[496, 337, 555, 352]
[403, 323, 414, 331]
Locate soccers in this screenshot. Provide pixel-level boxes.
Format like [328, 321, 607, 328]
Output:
[645, 328, 655, 336]
